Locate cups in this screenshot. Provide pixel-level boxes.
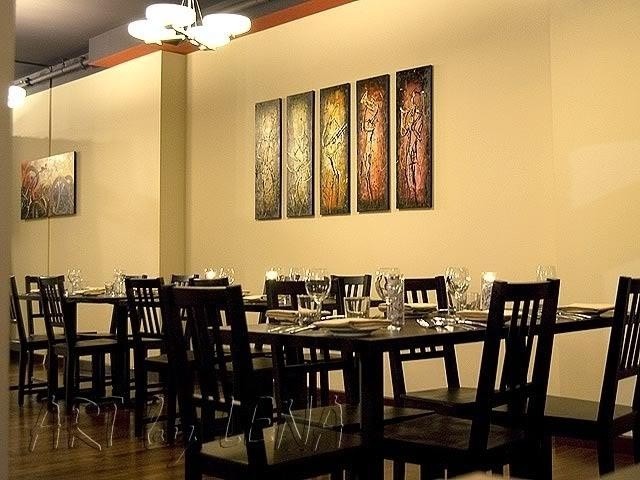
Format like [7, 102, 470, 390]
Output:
[480, 272, 497, 310]
[344, 297, 371, 318]
[536, 264, 557, 283]
[205, 267, 235, 286]
[296, 294, 323, 328]
[104, 281, 115, 295]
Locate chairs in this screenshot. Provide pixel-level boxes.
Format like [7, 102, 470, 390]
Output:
[10, 272, 640, 480]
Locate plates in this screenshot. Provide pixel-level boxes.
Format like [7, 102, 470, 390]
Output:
[323, 326, 381, 336]
[242, 295, 268, 301]
[402, 302, 439, 309]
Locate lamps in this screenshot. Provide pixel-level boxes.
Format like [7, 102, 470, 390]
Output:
[127, 0, 252, 51]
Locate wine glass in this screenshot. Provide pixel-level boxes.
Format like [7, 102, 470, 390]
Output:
[68, 266, 80, 296]
[306, 268, 331, 321]
[375, 267, 402, 322]
[445, 265, 471, 321]
[113, 264, 127, 296]
